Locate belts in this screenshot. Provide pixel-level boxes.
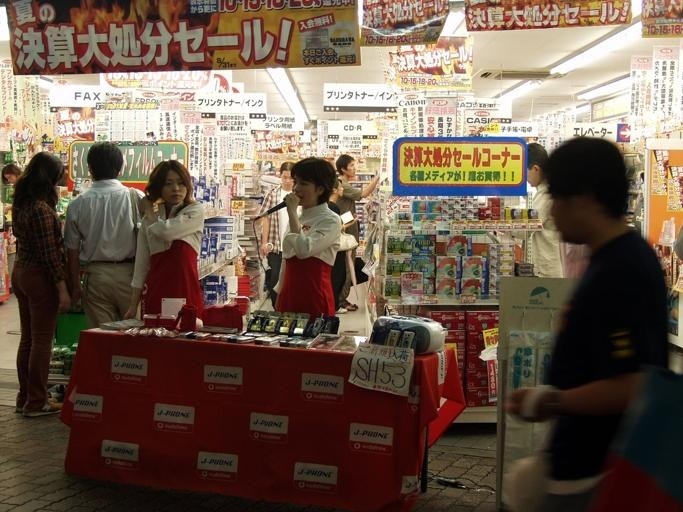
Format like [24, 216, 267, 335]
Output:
[91, 258, 133, 264]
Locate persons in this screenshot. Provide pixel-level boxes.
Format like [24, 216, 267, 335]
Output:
[64, 142, 146, 327]
[259, 154, 380, 320]
[1, 164, 22, 185]
[12, 152, 72, 417]
[504, 137, 670, 512]
[524, 142, 568, 279]
[125, 161, 205, 320]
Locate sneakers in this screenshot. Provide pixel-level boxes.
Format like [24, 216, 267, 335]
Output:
[334, 301, 359, 314]
[15, 392, 62, 417]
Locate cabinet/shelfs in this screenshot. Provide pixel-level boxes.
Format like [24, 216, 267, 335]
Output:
[242, 196, 268, 300]
[348, 172, 376, 259]
[194, 196, 241, 305]
[381, 227, 546, 424]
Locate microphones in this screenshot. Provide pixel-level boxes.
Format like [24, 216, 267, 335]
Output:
[255, 198, 289, 221]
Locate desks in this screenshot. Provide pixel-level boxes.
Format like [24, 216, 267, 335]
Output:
[60, 328, 466, 512]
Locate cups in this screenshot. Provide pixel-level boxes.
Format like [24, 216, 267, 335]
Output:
[175, 304, 197, 331]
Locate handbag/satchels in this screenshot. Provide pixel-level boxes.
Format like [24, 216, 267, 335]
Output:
[350, 257, 369, 286]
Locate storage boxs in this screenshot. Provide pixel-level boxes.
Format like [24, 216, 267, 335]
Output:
[429, 311, 500, 407]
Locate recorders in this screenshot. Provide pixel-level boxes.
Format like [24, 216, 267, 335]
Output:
[371, 315, 444, 354]
